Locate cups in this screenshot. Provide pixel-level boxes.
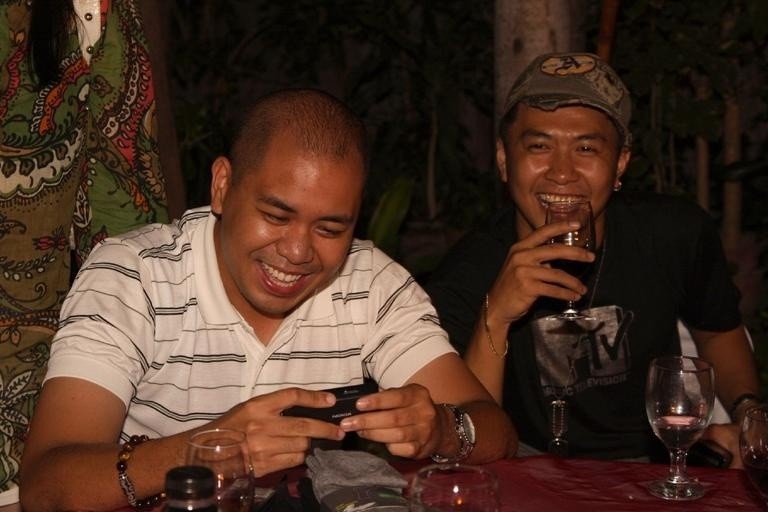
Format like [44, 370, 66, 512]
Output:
[183, 427, 256, 511]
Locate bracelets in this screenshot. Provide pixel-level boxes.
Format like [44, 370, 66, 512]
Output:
[481, 295, 511, 358]
[728, 390, 760, 416]
[116, 431, 170, 508]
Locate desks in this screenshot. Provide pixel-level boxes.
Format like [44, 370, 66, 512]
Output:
[114, 455, 768, 512]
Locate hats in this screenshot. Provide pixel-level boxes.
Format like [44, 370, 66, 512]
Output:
[501, 50, 634, 148]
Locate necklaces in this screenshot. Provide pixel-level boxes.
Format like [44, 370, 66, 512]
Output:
[537, 235, 606, 457]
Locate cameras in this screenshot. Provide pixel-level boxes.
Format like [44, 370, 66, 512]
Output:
[283, 384, 378, 441]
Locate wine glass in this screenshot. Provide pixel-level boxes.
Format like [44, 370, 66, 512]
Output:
[544, 199, 598, 323]
[644, 355, 718, 503]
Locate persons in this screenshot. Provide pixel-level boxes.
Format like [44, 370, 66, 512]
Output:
[0, 1, 186, 512]
[418, 45, 768, 471]
[16, 84, 518, 511]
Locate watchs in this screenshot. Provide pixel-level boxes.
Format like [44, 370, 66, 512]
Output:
[429, 399, 478, 466]
